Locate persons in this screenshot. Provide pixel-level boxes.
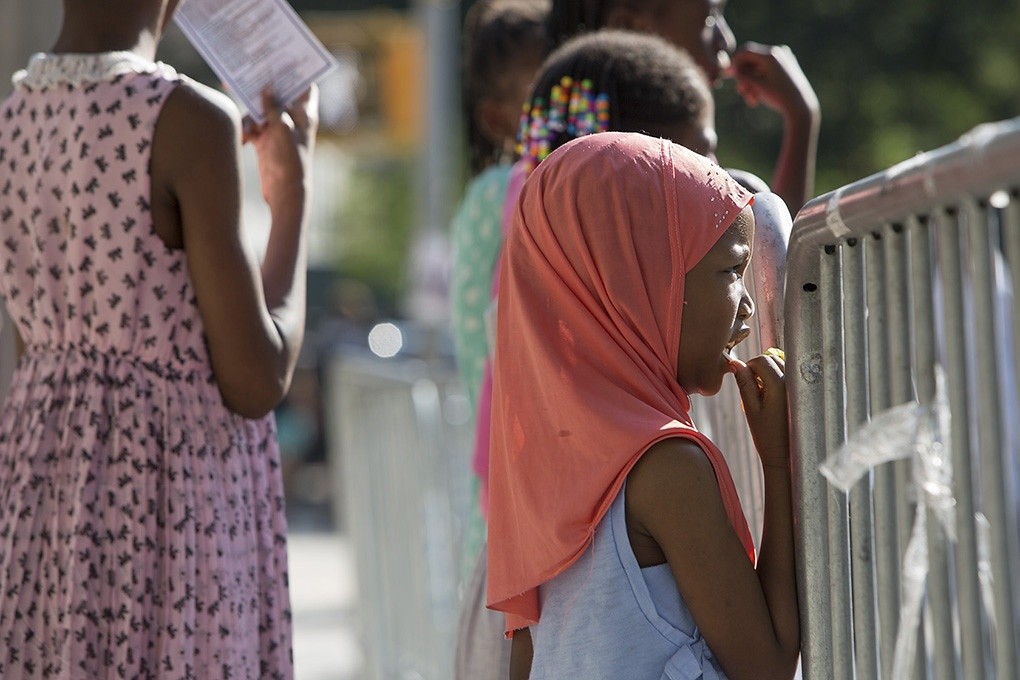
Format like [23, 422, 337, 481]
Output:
[2, 0, 323, 680]
[438, 0, 829, 680]
